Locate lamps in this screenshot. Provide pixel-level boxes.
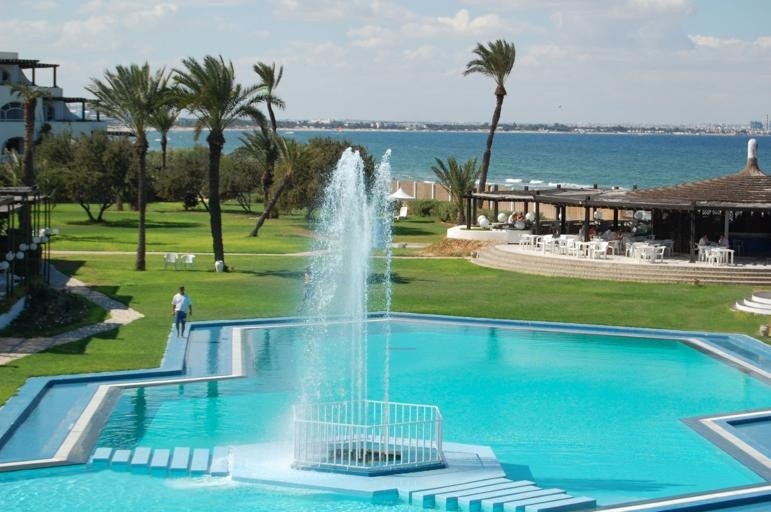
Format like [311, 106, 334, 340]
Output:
[163, 253, 178, 271]
[519, 228, 734, 265]
[395, 206, 408, 220]
[181, 252, 195, 271]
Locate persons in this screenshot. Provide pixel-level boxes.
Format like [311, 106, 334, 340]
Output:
[718, 234, 729, 249]
[699, 233, 711, 246]
[579, 222, 624, 243]
[517, 212, 524, 221]
[508, 212, 516, 224]
[297, 266, 314, 312]
[172, 286, 192, 338]
